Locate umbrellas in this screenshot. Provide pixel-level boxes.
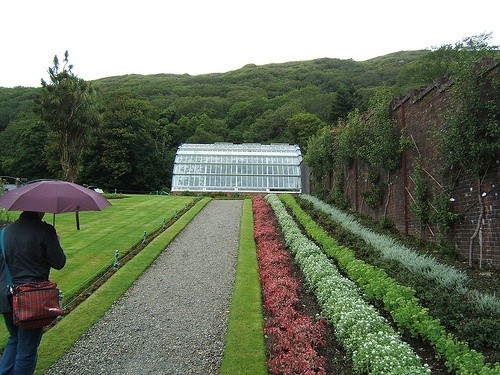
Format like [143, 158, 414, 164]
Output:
[0, 180, 113, 228]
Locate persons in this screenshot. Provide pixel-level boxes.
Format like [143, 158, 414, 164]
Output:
[0, 210, 69, 375]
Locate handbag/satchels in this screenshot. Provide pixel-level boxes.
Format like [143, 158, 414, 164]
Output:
[7, 281, 64, 330]
